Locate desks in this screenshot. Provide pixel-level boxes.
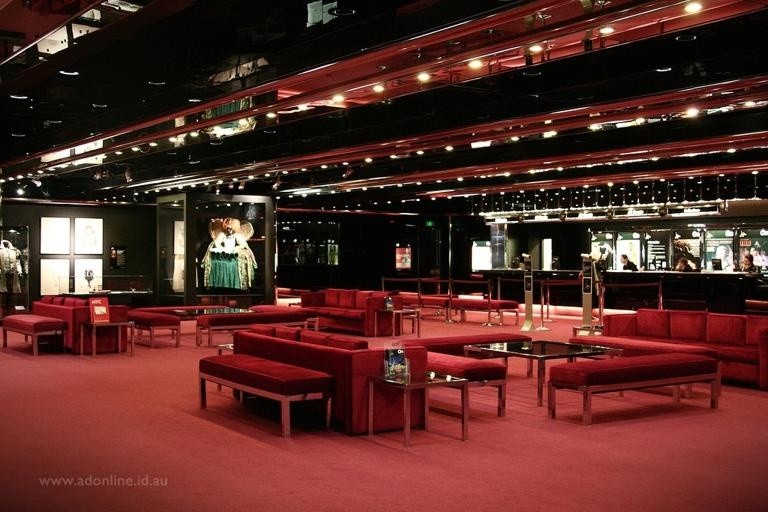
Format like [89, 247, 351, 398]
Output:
[172, 307, 255, 320]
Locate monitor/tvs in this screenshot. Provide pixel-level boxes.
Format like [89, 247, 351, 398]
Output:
[606, 253, 611, 270]
[652, 256, 656, 270]
[712, 259, 721, 270]
[512, 258, 519, 268]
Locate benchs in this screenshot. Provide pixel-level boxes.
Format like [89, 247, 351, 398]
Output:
[426, 351, 506, 421]
[198, 353, 335, 438]
[2, 314, 67, 355]
[396, 293, 521, 326]
[401, 333, 532, 378]
[196, 308, 321, 347]
[136, 306, 231, 322]
[545, 353, 722, 425]
[128, 309, 182, 349]
[249, 305, 320, 331]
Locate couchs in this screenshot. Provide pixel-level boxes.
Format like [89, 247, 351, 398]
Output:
[569, 314, 768, 391]
[301, 291, 404, 336]
[32, 300, 128, 354]
[232, 331, 428, 436]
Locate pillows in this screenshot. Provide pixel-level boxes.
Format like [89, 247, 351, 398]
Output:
[250, 323, 369, 351]
[324, 289, 394, 309]
[40, 294, 88, 307]
[635, 308, 768, 347]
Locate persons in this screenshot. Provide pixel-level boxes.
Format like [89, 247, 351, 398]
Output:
[675, 257, 694, 273]
[619, 253, 638, 271]
[510, 255, 525, 269]
[732, 255, 761, 273]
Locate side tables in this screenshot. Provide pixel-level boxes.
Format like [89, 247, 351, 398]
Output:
[367, 371, 469, 447]
[374, 309, 421, 338]
[80, 321, 135, 355]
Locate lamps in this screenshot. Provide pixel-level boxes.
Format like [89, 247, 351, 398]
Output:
[0, 0, 768, 228]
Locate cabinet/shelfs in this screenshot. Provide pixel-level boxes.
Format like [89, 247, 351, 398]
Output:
[58, 275, 153, 310]
